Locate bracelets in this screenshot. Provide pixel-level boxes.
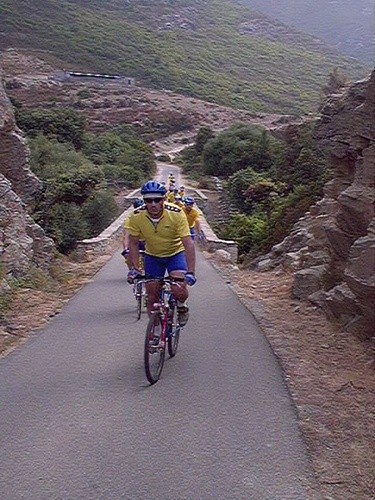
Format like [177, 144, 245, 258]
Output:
[186, 272, 193, 274]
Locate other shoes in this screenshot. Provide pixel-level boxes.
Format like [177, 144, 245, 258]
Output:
[176, 300, 190, 326]
[127, 272, 134, 284]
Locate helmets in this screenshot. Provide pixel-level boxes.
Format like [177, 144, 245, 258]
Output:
[169, 172, 185, 198]
[134, 200, 144, 208]
[184, 197, 194, 204]
[141, 181, 167, 197]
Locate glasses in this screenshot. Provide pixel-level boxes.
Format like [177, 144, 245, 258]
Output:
[143, 198, 164, 203]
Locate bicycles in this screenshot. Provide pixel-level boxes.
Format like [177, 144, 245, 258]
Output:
[132, 276, 187, 384]
[120, 249, 148, 319]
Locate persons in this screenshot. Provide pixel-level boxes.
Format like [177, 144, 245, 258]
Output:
[127, 180, 196, 352]
[124, 198, 147, 307]
[167, 173, 185, 207]
[183, 197, 199, 241]
